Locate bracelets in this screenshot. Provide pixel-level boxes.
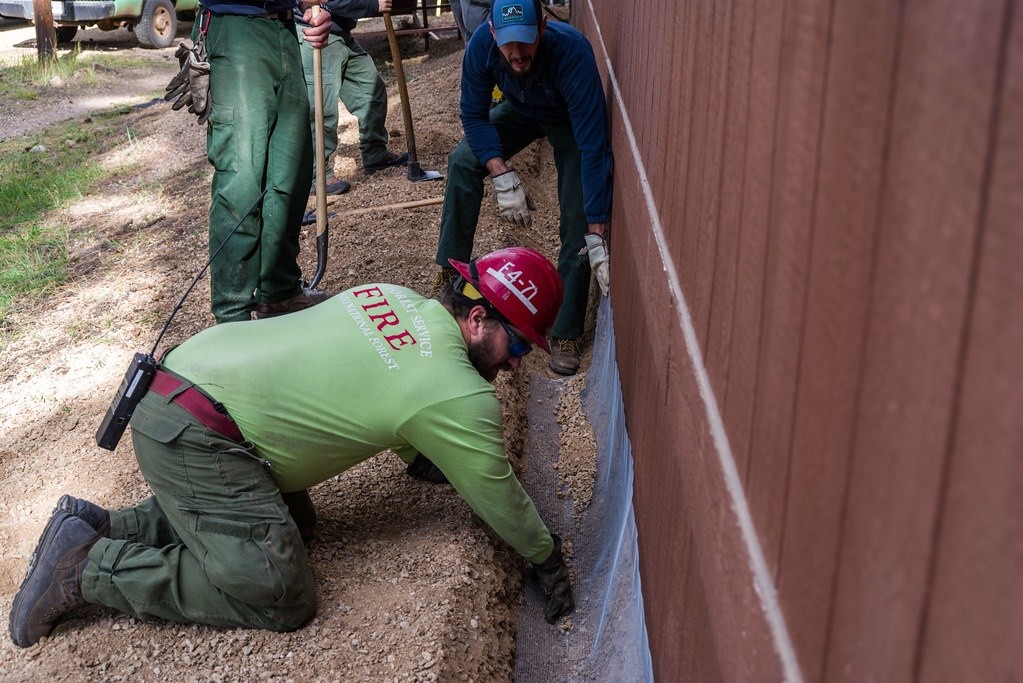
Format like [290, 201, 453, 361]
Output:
[318, 3, 331, 13]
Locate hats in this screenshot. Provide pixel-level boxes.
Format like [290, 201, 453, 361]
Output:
[493, 0, 542, 47]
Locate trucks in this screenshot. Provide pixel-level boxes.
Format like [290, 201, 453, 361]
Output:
[0, 1, 202, 50]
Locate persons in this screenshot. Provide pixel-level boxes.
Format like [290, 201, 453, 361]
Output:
[429, 0, 614, 375]
[9, 246, 574, 648]
[190, 1, 333, 325]
[449, 0, 492, 47]
[292, 0, 409, 196]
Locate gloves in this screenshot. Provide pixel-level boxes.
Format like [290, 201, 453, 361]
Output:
[584, 230, 609, 297]
[491, 168, 536, 228]
[406, 451, 450, 484]
[529, 533, 574, 623]
[164, 31, 212, 125]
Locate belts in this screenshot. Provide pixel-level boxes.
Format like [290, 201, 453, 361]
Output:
[148, 371, 245, 444]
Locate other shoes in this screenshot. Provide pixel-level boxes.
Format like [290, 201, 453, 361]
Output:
[256, 287, 333, 318]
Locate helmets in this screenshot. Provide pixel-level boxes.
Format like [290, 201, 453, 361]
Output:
[448, 247, 564, 355]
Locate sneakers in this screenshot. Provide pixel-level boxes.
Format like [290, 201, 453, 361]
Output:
[429, 266, 461, 300]
[364, 152, 409, 174]
[549, 334, 581, 375]
[313, 175, 351, 196]
[9, 509, 101, 648]
[52, 493, 110, 540]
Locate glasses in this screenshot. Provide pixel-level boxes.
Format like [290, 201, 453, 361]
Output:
[492, 311, 533, 358]
